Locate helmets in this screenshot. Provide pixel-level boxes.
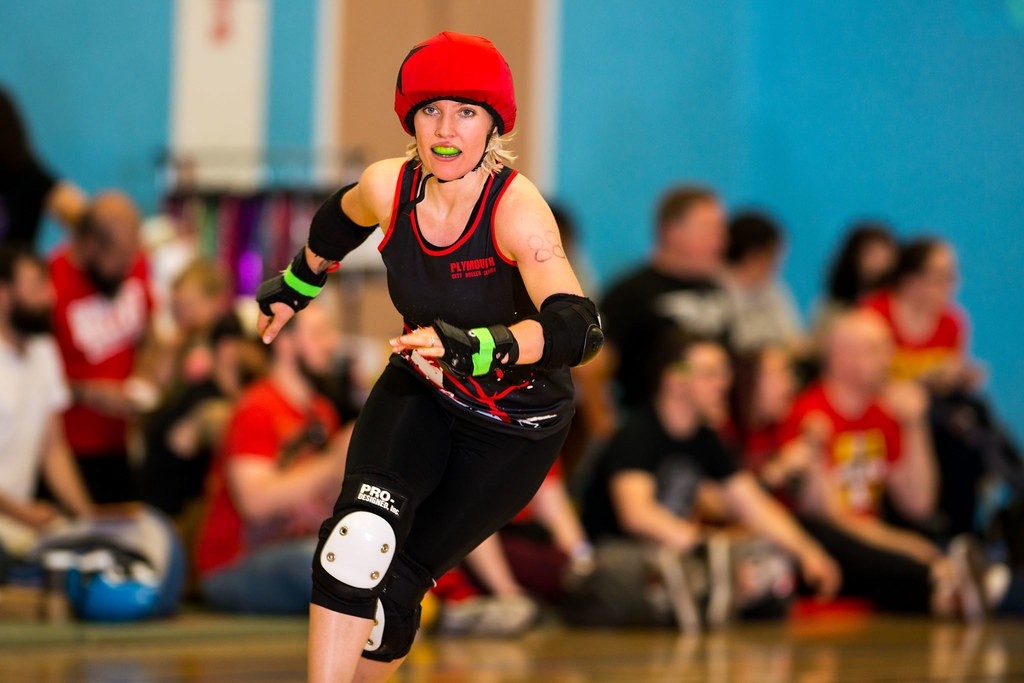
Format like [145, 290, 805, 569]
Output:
[394, 30, 516, 137]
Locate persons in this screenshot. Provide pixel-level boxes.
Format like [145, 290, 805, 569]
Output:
[0, 85, 1024, 683]
[254, 32, 606, 683]
